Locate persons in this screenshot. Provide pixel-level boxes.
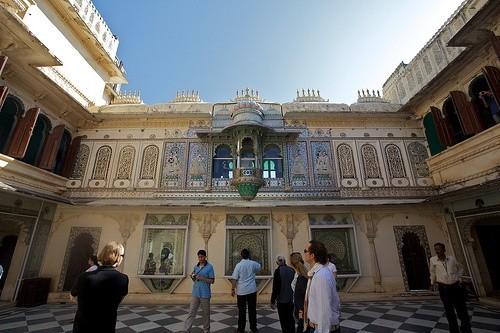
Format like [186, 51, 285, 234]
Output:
[71, 241, 129, 333]
[182, 250, 215, 333]
[231, 249, 263, 333]
[142, 253, 170, 275]
[479, 89, 500, 123]
[85, 256, 100, 273]
[271, 240, 341, 333]
[430, 243, 473, 333]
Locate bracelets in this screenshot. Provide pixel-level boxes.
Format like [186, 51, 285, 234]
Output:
[231, 289, 235, 290]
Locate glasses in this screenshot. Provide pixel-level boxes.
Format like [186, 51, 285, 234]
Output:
[120, 254, 124, 259]
[304, 249, 309, 253]
